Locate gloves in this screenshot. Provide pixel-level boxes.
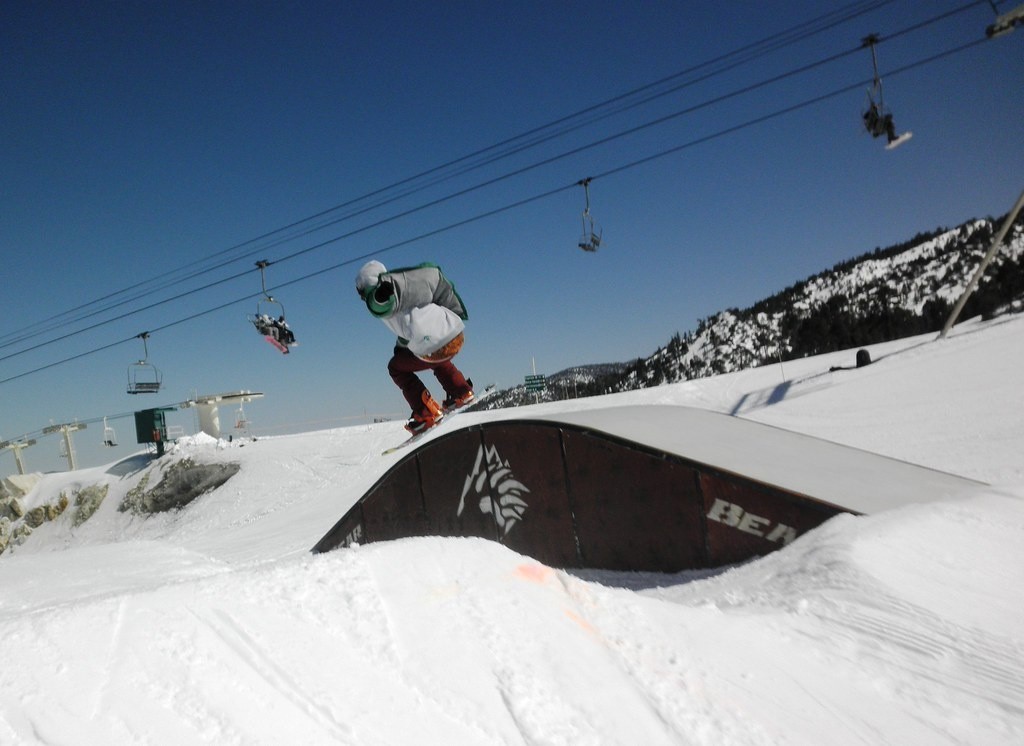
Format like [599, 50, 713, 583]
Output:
[376, 280, 394, 303]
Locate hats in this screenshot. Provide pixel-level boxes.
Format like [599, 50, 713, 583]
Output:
[355, 259, 386, 300]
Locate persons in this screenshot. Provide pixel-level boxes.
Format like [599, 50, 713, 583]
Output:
[255, 314, 298, 348]
[353, 257, 472, 438]
[863, 102, 910, 143]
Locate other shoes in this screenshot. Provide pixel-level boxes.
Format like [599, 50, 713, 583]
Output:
[404, 397, 444, 430]
[442, 381, 475, 411]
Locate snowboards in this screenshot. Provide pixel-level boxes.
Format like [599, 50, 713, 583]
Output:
[380, 383, 497, 456]
